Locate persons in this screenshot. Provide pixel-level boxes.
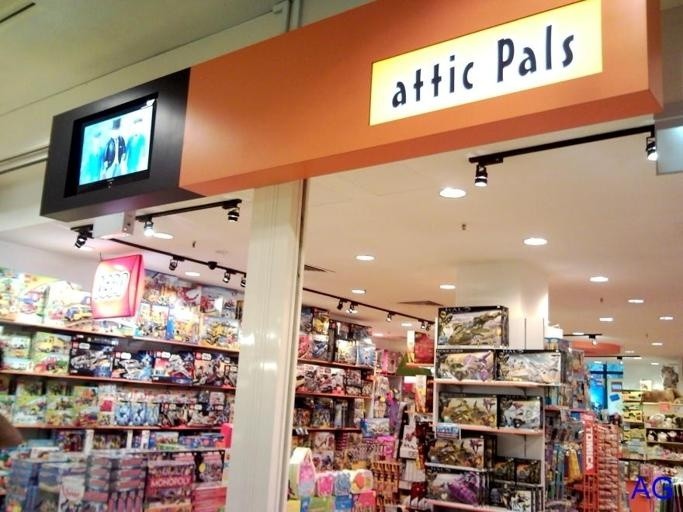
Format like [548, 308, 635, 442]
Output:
[103, 117, 126, 179]
[124, 118, 149, 174]
[0, 270, 381, 510]
[79, 131, 106, 185]
[380, 304, 683, 512]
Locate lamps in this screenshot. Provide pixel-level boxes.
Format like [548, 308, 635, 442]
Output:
[165, 249, 435, 333]
[456, 124, 659, 188]
[71, 192, 241, 253]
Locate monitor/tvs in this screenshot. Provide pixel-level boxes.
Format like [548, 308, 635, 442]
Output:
[62, 92, 160, 199]
